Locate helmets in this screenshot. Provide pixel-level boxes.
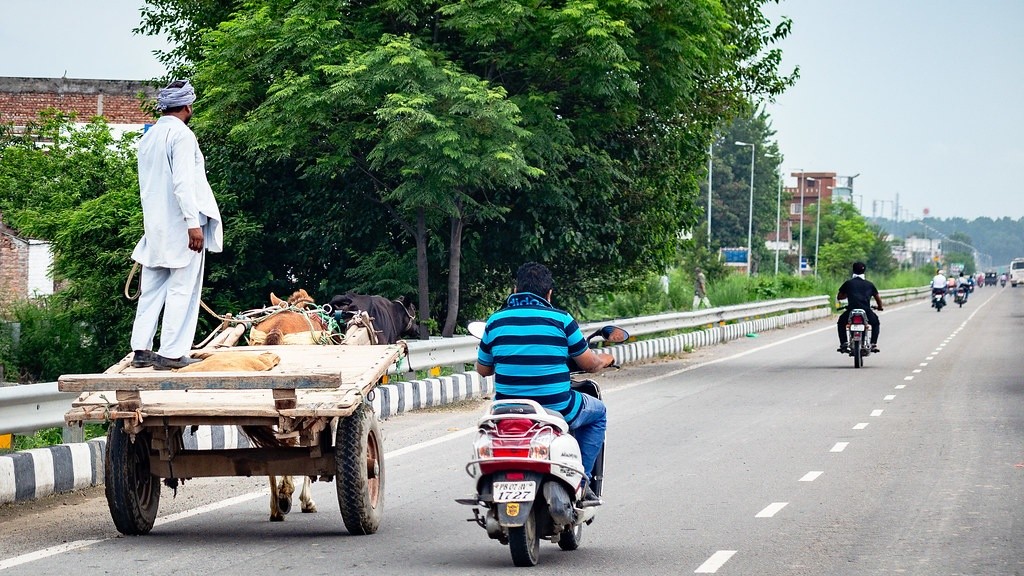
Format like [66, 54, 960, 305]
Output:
[938, 270, 944, 275]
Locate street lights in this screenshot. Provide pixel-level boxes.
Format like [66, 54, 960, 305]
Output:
[734, 141, 755, 280]
[792, 167, 804, 277]
[850, 193, 863, 215]
[764, 153, 781, 275]
[807, 177, 820, 279]
[918, 222, 992, 274]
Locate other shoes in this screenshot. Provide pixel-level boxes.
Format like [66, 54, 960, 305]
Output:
[839, 343, 848, 350]
[582, 485, 599, 501]
[932, 304, 934, 307]
[871, 346, 880, 353]
[153, 354, 202, 371]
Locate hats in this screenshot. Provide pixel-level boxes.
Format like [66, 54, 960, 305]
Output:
[852, 262, 867, 275]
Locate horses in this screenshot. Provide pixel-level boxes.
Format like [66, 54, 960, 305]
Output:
[251, 289, 424, 519]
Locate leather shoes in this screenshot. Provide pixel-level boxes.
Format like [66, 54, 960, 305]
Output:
[132, 350, 157, 367]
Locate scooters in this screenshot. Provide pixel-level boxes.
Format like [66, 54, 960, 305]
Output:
[454, 322, 628, 568]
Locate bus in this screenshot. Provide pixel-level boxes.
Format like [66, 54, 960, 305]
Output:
[1008, 261, 1024, 287]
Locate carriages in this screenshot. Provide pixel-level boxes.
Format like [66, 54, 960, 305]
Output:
[58, 290, 421, 537]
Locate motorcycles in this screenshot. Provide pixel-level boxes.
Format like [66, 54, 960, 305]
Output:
[930, 281, 983, 313]
[984, 272, 997, 287]
[836, 305, 883, 367]
[1000, 281, 1005, 288]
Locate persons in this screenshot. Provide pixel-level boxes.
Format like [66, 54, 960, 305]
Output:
[1000, 273, 1007, 285]
[603, 326, 628, 341]
[478, 265, 615, 501]
[659, 275, 668, 295]
[130, 80, 222, 369]
[837, 262, 883, 351]
[931, 269, 956, 307]
[955, 272, 983, 302]
[693, 267, 712, 308]
